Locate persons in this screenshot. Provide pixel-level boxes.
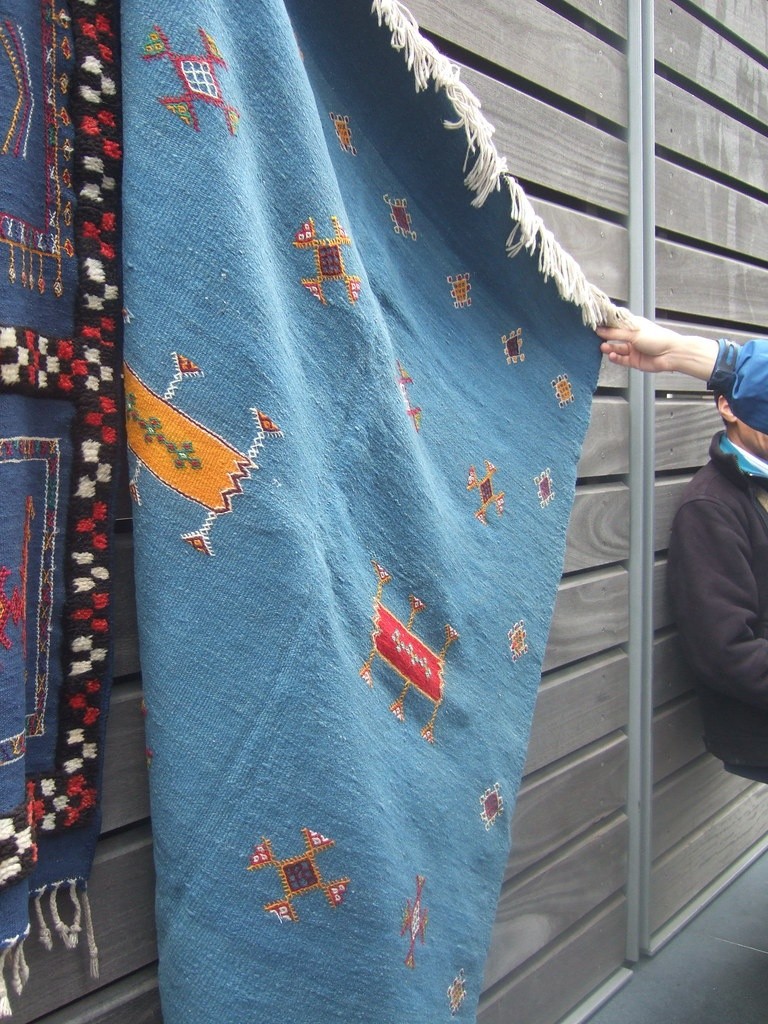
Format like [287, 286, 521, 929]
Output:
[595, 315, 768, 436]
[666, 391, 768, 785]
